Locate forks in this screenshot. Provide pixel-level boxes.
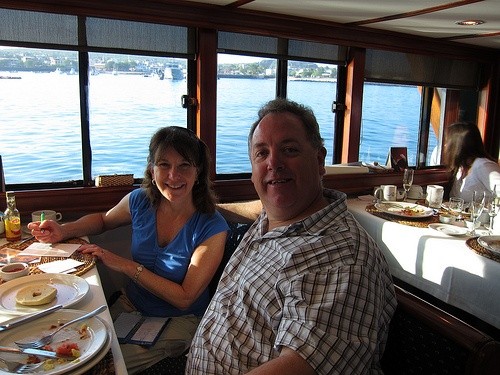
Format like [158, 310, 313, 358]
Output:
[14, 304, 108, 351]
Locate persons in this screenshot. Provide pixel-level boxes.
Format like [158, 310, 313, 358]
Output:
[444, 122, 500, 204]
[185, 98, 398, 375]
[27, 125, 231, 375]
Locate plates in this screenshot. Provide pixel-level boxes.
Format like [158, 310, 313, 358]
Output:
[476, 235, 500, 254]
[442, 200, 480, 215]
[0, 308, 117, 375]
[374, 201, 434, 217]
[0, 273, 90, 319]
[428, 222, 468, 236]
[0, 262, 30, 282]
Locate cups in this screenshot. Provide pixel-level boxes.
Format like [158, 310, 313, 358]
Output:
[408, 185, 423, 199]
[32, 209, 62, 226]
[373, 185, 397, 202]
[471, 211, 490, 225]
[424, 185, 444, 209]
[448, 197, 464, 219]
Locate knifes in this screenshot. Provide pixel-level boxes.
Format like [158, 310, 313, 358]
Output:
[0, 345, 78, 361]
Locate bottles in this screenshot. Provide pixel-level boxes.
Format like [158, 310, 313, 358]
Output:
[3, 190, 22, 242]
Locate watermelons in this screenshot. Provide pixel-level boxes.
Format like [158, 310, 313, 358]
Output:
[56, 342, 78, 356]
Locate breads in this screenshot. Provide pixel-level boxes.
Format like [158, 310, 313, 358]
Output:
[15, 284, 58, 305]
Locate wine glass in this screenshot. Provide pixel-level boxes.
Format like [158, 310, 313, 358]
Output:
[484, 190, 500, 236]
[403, 169, 414, 200]
[466, 190, 487, 237]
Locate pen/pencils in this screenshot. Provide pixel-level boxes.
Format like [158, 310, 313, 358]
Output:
[40, 211, 46, 234]
[18, 235, 35, 246]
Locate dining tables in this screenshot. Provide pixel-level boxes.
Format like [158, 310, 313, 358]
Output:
[0, 222, 128, 375]
[216, 197, 500, 375]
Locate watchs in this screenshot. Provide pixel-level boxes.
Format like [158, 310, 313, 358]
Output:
[134, 264, 144, 283]
